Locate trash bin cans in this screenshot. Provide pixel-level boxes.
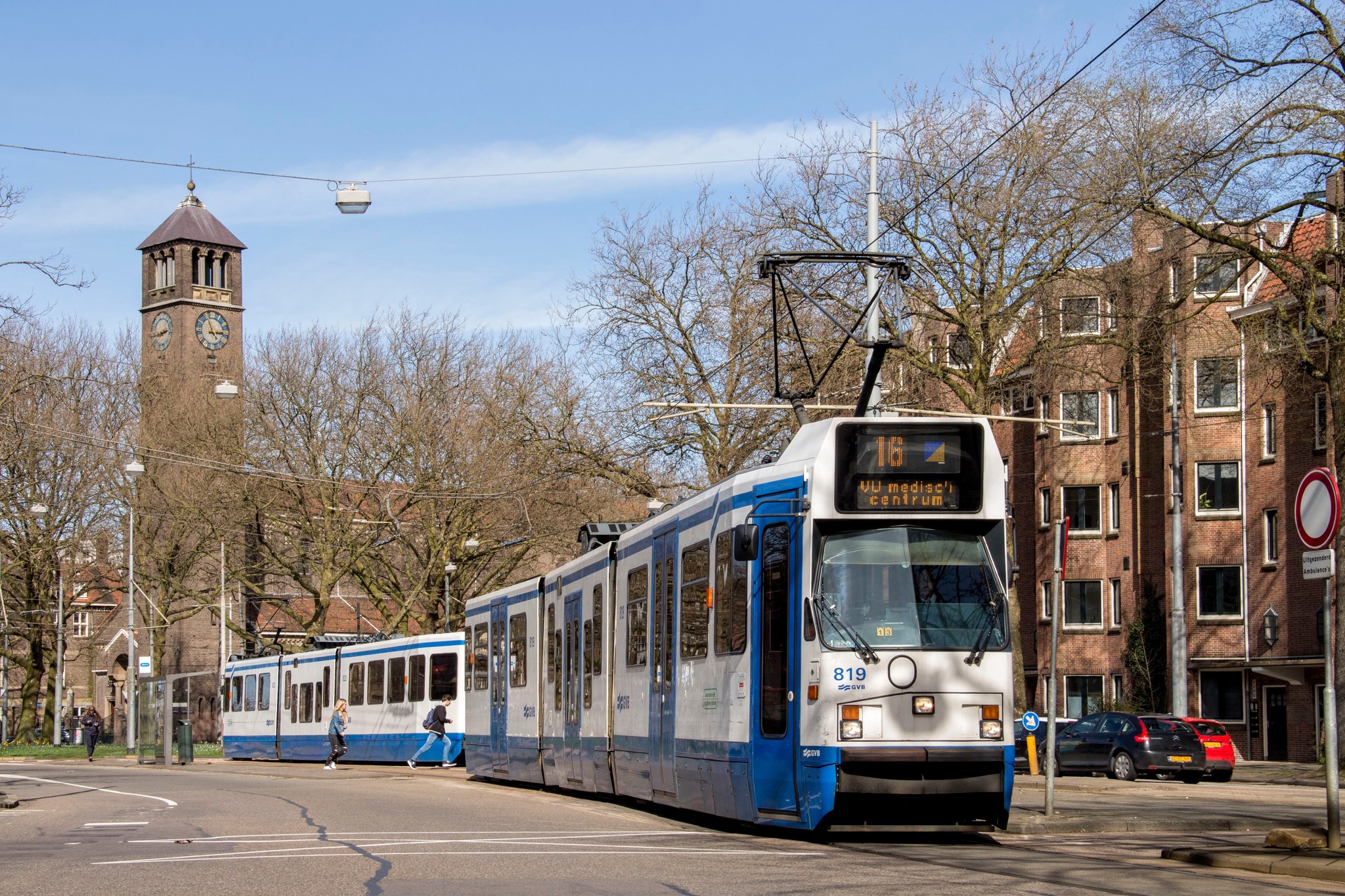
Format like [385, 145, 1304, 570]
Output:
[177, 720, 194, 766]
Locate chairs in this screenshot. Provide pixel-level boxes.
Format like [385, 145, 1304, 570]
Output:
[1110, 722, 1123, 732]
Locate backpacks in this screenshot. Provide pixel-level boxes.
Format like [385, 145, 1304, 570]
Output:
[422, 708, 439, 730]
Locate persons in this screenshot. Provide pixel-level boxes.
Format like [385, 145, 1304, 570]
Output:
[81, 705, 102, 762]
[407, 694, 457, 770]
[324, 699, 349, 769]
[906, 574, 943, 604]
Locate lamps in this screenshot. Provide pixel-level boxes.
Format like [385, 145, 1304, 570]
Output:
[464, 537, 479, 550]
[444, 563, 458, 573]
[124, 460, 145, 477]
[30, 503, 47, 517]
[334, 182, 371, 215]
[213, 380, 239, 400]
[645, 498, 665, 511]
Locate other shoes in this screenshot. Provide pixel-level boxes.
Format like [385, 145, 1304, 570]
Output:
[89, 756, 93, 762]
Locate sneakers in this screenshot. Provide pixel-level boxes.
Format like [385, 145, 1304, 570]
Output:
[323, 766, 332, 770]
[330, 761, 336, 769]
[442, 760, 457, 767]
[407, 759, 418, 770]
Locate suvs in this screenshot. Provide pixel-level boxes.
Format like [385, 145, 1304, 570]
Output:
[1034, 710, 1207, 785]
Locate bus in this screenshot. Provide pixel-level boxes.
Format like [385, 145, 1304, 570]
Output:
[463, 246, 1017, 833]
[223, 630, 469, 765]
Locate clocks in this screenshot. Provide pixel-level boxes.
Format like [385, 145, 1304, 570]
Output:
[148, 312, 173, 351]
[194, 308, 230, 351]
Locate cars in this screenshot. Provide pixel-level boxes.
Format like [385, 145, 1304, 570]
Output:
[1012, 716, 1094, 773]
[1156, 716, 1236, 782]
